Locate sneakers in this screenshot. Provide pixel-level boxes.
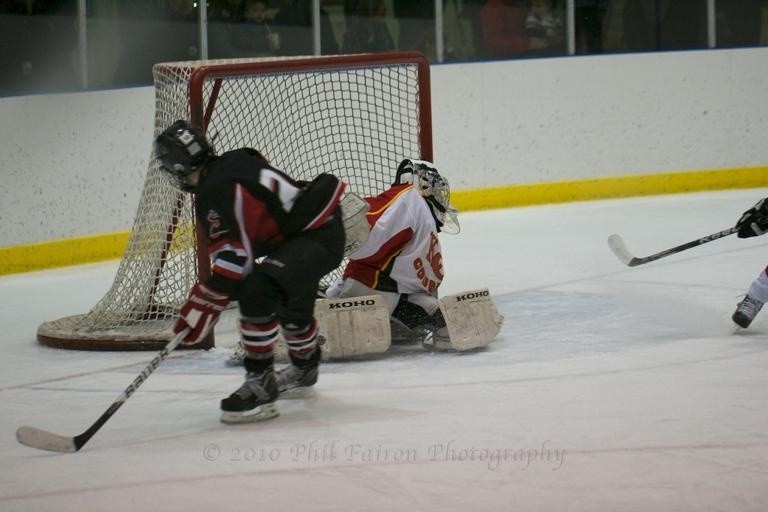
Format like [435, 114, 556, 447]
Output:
[223, 360, 278, 409]
[732, 295, 763, 328]
[278, 353, 318, 392]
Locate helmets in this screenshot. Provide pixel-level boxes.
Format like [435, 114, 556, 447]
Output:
[154, 120, 213, 193]
[395, 158, 461, 232]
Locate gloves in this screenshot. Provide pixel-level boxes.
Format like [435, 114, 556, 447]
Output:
[173, 281, 232, 345]
[736, 198, 767, 238]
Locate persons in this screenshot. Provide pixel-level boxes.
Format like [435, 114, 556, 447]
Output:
[161, 0, 767, 84]
[731, 196, 767, 330]
[223, 159, 453, 366]
[154, 116, 347, 413]
